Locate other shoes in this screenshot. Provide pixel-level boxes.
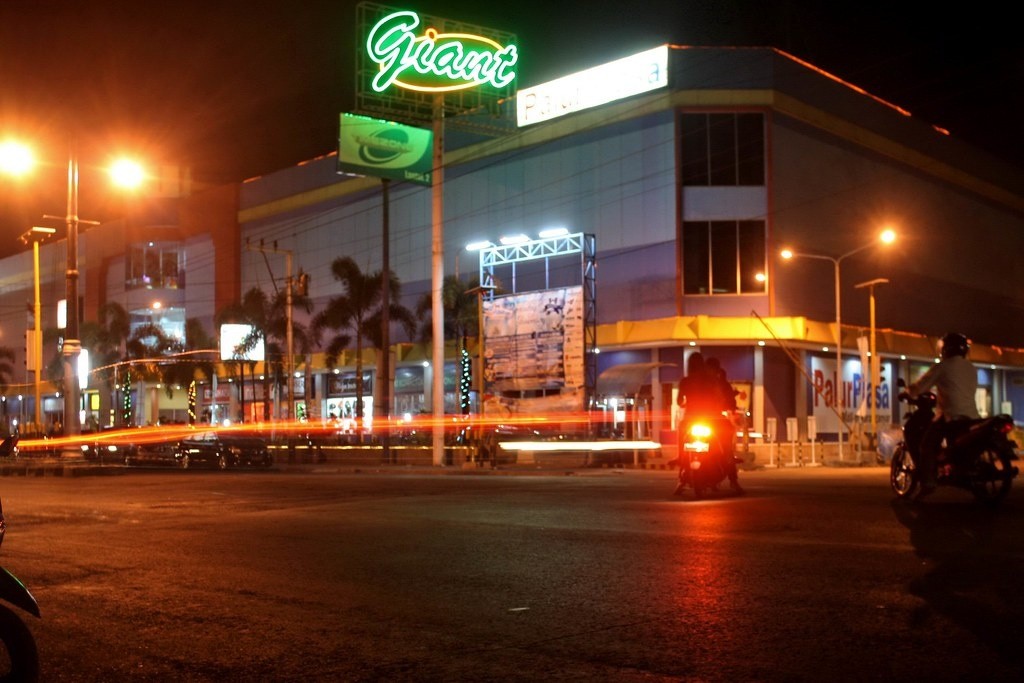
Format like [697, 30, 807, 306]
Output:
[730, 481, 745, 494]
[909, 481, 935, 503]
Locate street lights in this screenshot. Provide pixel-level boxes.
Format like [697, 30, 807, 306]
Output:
[17, 226, 57, 429]
[0, 136, 149, 477]
[779, 229, 897, 462]
[854, 277, 892, 449]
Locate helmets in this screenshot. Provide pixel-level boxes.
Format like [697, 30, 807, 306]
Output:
[942, 331, 970, 358]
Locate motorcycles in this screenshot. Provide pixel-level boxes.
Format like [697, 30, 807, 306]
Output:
[889, 376, 1021, 505]
[678, 403, 730, 499]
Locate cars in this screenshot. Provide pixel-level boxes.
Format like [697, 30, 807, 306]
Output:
[173, 430, 272, 472]
[80, 439, 142, 462]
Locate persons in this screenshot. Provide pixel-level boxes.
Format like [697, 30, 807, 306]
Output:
[671, 357, 743, 496]
[676, 352, 726, 467]
[897, 333, 983, 506]
